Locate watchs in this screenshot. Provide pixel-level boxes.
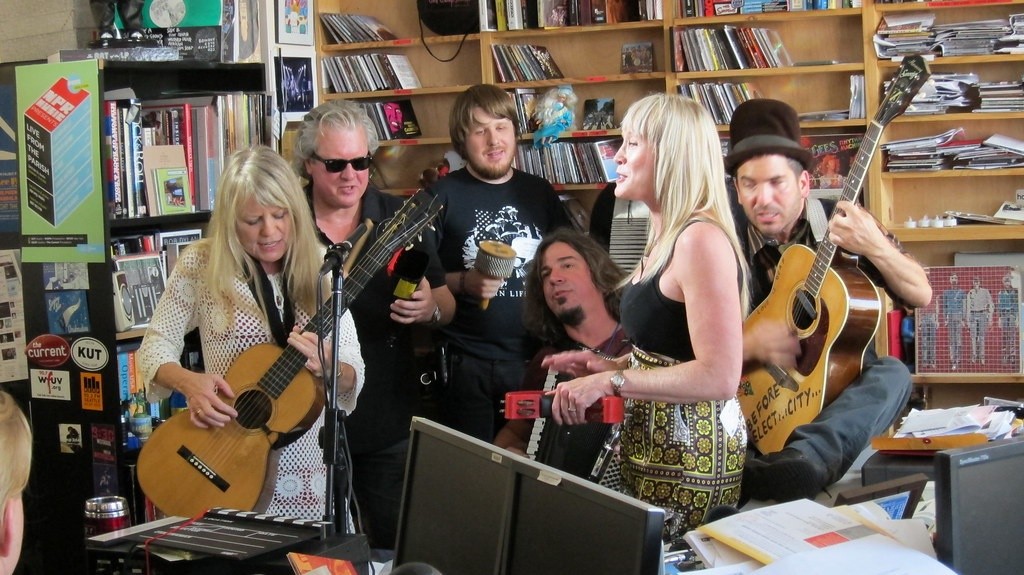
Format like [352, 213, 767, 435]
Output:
[425, 304, 441, 327]
[610, 368, 625, 398]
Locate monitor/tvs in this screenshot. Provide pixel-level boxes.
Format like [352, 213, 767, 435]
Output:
[393, 415, 664, 575]
[933, 436, 1024, 575]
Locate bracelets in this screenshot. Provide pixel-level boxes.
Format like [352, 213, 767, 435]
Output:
[338, 361, 343, 378]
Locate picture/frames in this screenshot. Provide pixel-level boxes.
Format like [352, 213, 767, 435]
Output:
[274, 0, 315, 46]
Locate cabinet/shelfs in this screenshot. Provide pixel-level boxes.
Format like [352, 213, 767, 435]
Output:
[1, 0, 1024, 469]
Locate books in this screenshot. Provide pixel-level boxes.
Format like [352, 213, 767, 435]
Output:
[480, 0, 663, 187]
[320, 14, 423, 140]
[104, 91, 275, 439]
[675, 0, 864, 178]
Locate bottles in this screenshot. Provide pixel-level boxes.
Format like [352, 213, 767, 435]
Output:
[119, 391, 153, 451]
[96, 559, 143, 575]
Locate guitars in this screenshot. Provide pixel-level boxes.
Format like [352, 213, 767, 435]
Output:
[737, 53, 933, 454]
[137, 188, 444, 515]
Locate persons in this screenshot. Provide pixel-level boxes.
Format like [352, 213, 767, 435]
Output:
[89, 0, 145, 39]
[0, 390, 32, 575]
[528, 86, 576, 150]
[131, 144, 365, 533]
[293, 83, 933, 563]
[543, 93, 749, 544]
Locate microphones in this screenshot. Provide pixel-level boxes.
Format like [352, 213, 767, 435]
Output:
[321, 223, 368, 276]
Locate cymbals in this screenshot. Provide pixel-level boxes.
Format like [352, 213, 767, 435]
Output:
[499, 390, 632, 424]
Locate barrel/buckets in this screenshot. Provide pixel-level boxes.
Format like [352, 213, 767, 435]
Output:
[83, 496, 131, 546]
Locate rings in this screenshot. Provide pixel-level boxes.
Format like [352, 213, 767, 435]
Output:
[196, 409, 202, 414]
[568, 409, 576, 412]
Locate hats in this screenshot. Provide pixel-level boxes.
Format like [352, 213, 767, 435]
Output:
[721, 97, 812, 171]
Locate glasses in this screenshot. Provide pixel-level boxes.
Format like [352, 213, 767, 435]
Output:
[311, 150, 374, 174]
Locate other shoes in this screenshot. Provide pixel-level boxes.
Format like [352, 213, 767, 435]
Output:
[742, 444, 820, 499]
